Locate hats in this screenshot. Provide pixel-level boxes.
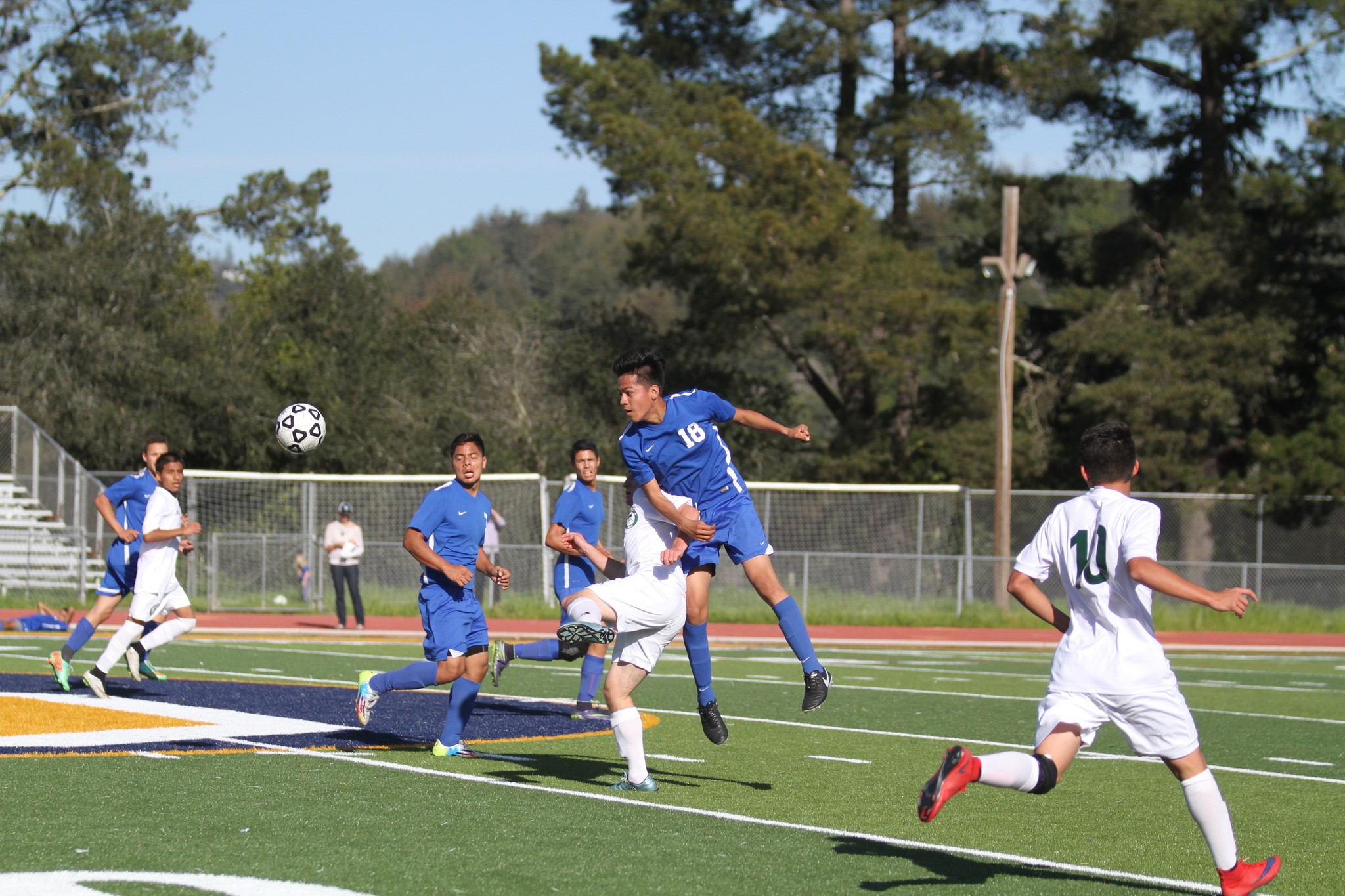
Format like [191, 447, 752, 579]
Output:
[338, 502, 353, 517]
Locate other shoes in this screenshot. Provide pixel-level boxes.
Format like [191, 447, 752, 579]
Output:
[337, 623, 345, 629]
[358, 624, 364, 630]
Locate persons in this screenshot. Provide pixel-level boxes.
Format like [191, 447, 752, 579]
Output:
[355, 432, 511, 758]
[916, 421, 1282, 896]
[473, 509, 507, 604]
[557, 469, 700, 793]
[48, 433, 189, 691]
[82, 452, 202, 699]
[323, 502, 365, 630]
[489, 438, 611, 721]
[615, 351, 833, 745]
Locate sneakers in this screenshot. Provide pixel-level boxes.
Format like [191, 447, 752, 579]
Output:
[697, 697, 728, 745]
[432, 738, 483, 757]
[801, 667, 833, 712]
[48, 651, 73, 691]
[139, 660, 168, 681]
[1217, 854, 1282, 896]
[355, 670, 379, 725]
[124, 645, 141, 682]
[918, 745, 973, 822]
[570, 703, 611, 721]
[82, 670, 110, 699]
[609, 771, 659, 792]
[557, 621, 614, 644]
[490, 640, 510, 687]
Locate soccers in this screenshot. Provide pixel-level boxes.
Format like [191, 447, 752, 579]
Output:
[275, 403, 327, 455]
[273, 592, 288, 606]
